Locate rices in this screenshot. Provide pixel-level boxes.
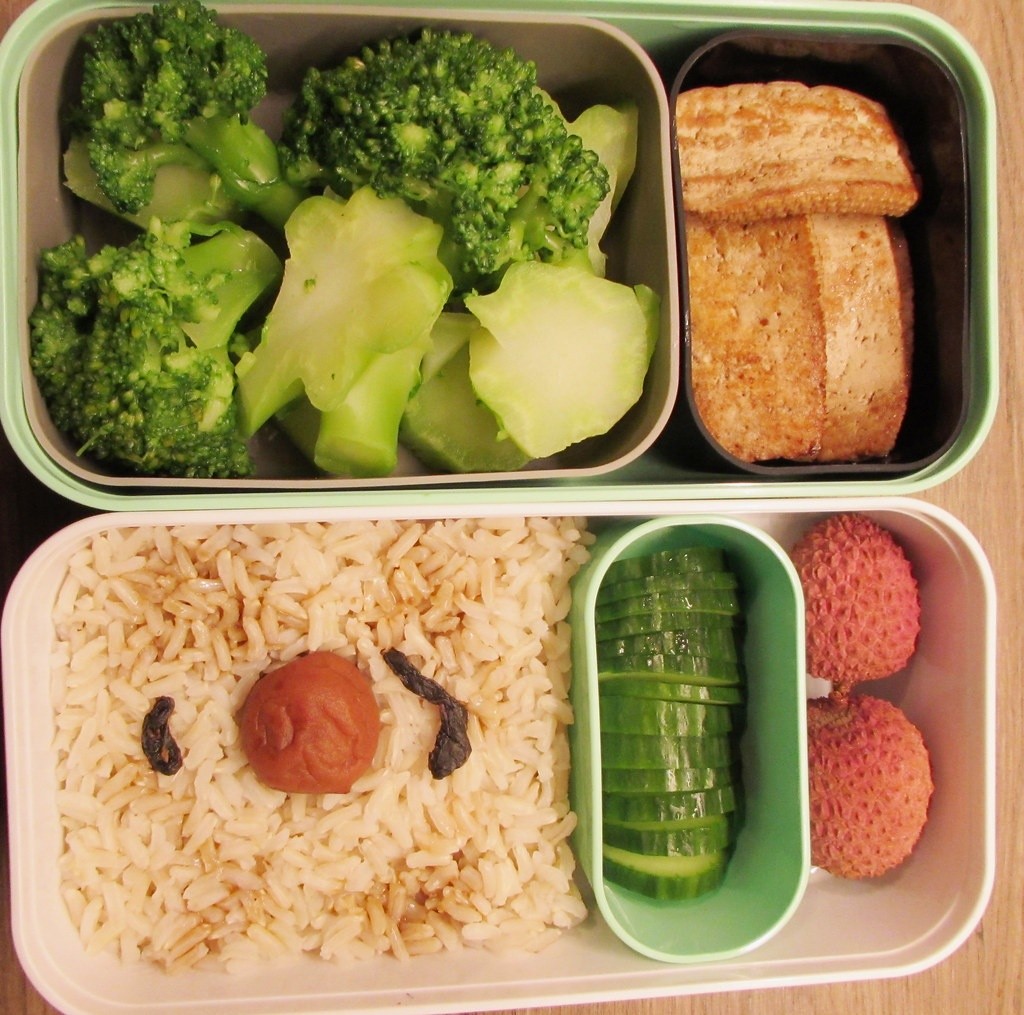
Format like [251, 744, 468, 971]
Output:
[42, 514, 607, 973]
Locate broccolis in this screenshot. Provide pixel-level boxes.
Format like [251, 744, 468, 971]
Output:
[25, 2, 663, 483]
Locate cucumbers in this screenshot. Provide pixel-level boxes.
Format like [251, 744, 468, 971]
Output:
[594, 542, 752, 907]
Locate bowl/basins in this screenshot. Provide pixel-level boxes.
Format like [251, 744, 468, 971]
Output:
[0, 1, 1001, 1012]
